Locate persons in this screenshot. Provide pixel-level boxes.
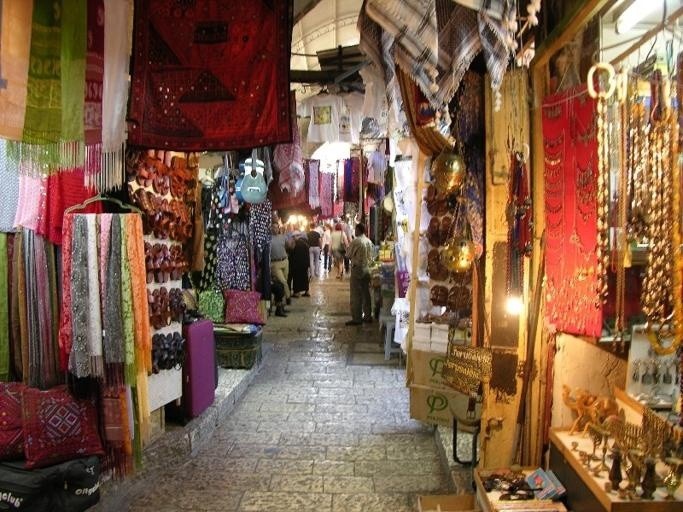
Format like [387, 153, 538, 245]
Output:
[269, 214, 376, 326]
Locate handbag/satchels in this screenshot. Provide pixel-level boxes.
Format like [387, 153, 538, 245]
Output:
[339, 244, 345, 253]
[441, 345, 492, 396]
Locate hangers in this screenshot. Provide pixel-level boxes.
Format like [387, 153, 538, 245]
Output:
[63, 186, 145, 220]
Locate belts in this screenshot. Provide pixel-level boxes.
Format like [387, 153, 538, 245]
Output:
[270, 257, 287, 261]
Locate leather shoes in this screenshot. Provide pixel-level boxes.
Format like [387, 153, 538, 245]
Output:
[280, 304, 289, 313]
[275, 306, 287, 317]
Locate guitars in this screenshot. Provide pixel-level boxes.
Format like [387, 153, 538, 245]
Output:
[236, 149, 267, 204]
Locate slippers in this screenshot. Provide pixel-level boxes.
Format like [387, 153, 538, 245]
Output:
[126, 148, 198, 376]
[414, 185, 470, 329]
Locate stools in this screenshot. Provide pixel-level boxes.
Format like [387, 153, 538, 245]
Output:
[214, 324, 262, 367]
[383, 322, 404, 361]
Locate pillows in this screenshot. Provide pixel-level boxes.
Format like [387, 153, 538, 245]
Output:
[0, 377, 106, 463]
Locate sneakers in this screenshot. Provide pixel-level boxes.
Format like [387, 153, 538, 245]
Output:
[345, 320, 362, 325]
[286, 298, 291, 304]
[328, 268, 331, 272]
[335, 275, 341, 279]
[324, 266, 327, 269]
[363, 316, 373, 322]
[301, 292, 311, 297]
[292, 292, 299, 296]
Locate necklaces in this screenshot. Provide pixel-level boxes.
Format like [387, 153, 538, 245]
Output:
[541, 63, 681, 356]
[504, 152, 535, 299]
